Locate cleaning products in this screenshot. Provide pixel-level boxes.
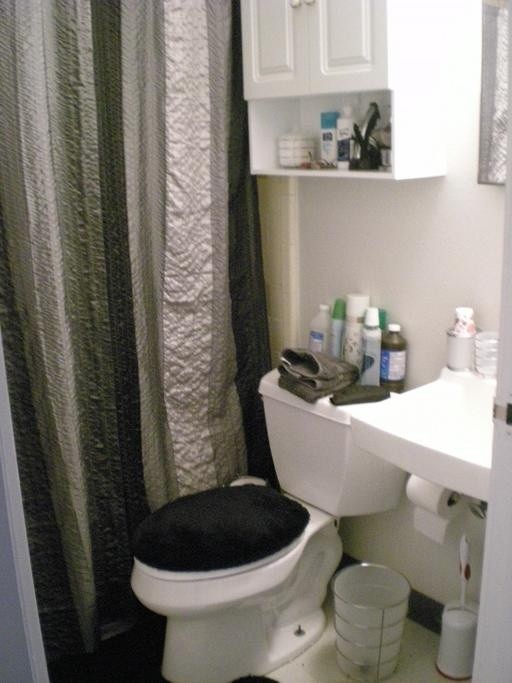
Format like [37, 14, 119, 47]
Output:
[308, 297, 408, 397]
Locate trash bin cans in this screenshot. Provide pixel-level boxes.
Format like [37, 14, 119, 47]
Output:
[328, 563, 413, 683]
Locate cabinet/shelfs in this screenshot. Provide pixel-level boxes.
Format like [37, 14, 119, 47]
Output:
[238, 0, 454, 183]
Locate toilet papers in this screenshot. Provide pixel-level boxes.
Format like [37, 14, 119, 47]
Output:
[403, 475, 470, 542]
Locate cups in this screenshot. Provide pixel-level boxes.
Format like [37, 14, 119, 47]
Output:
[474, 331, 498, 374]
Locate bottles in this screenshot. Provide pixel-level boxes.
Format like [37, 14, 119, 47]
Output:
[307, 291, 407, 394]
[278, 120, 314, 167]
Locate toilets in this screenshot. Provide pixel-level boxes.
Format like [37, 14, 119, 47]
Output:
[127, 363, 403, 683]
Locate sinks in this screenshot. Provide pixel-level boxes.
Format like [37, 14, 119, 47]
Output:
[350, 371, 494, 502]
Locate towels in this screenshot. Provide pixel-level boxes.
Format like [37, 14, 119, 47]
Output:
[278, 344, 358, 402]
